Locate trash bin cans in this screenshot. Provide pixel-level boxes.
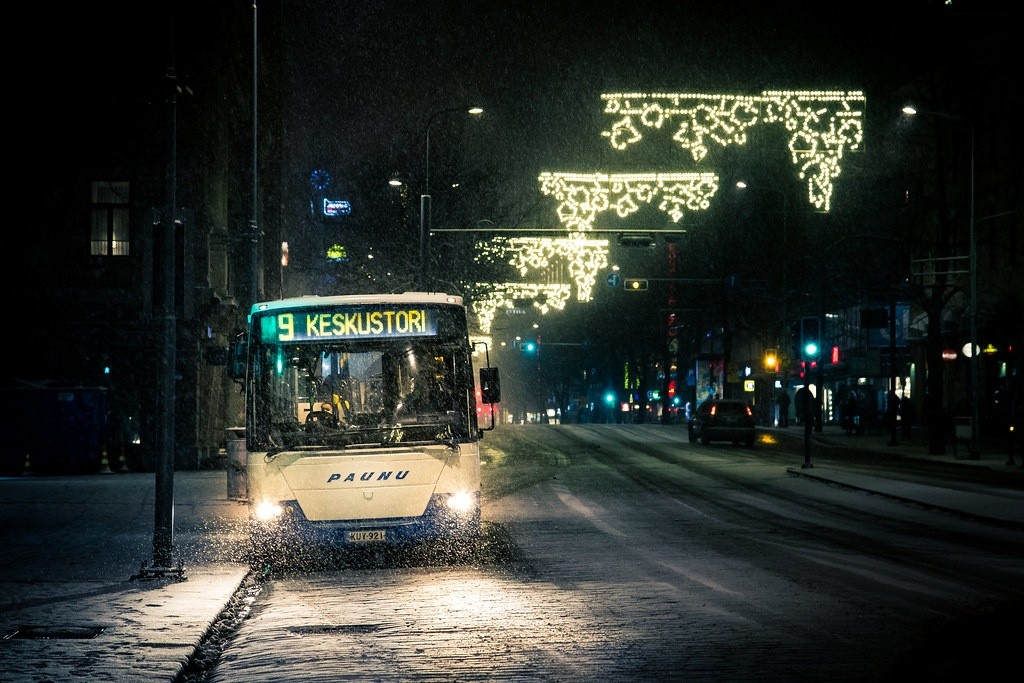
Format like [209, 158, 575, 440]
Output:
[223, 426, 247, 503]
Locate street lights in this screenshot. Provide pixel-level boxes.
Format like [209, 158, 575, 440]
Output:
[420, 104, 485, 292]
[901, 105, 980, 460]
[736, 181, 789, 428]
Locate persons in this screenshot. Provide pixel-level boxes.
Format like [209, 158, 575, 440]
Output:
[776, 389, 790, 429]
[708, 393, 720, 400]
[316, 403, 339, 431]
[396, 369, 453, 419]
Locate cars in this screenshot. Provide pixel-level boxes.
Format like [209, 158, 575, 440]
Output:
[688, 400, 756, 448]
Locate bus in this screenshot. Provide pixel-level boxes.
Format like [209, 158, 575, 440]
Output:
[228, 291, 501, 574]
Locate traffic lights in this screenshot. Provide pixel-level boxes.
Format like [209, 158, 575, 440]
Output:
[801, 316, 821, 362]
[519, 343, 536, 351]
[624, 280, 648, 291]
[765, 349, 778, 373]
[537, 335, 541, 344]
